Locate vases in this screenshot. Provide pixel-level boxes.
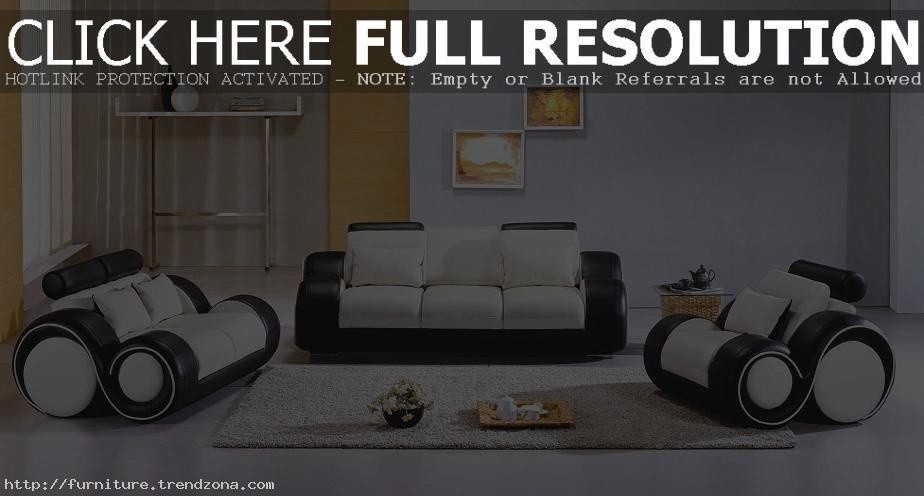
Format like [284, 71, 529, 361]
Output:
[160, 64, 199, 111]
[381, 409, 422, 428]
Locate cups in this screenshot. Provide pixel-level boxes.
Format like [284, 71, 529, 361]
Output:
[518, 403, 548, 422]
[679, 278, 690, 290]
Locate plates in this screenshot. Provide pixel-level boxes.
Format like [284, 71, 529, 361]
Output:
[170, 86, 199, 112]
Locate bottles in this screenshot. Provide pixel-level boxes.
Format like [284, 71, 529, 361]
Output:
[160, 64, 177, 112]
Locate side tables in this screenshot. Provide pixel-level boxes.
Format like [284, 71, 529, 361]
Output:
[652, 281, 733, 323]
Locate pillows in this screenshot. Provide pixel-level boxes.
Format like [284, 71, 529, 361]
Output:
[348, 238, 581, 290]
[89, 271, 186, 341]
[715, 268, 830, 343]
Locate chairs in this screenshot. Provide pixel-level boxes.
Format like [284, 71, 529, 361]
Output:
[12, 249, 282, 426]
[644, 254, 897, 430]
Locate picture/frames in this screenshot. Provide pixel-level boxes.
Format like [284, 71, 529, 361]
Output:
[451, 82, 586, 191]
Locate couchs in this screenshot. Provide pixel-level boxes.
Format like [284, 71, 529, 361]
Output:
[292, 219, 629, 363]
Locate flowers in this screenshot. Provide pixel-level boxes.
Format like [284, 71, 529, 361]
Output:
[364, 374, 436, 424]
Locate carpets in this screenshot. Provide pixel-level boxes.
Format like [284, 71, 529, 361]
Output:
[210, 359, 800, 452]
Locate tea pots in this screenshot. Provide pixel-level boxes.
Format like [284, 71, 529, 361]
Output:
[490, 392, 527, 421]
[688, 265, 715, 289]
